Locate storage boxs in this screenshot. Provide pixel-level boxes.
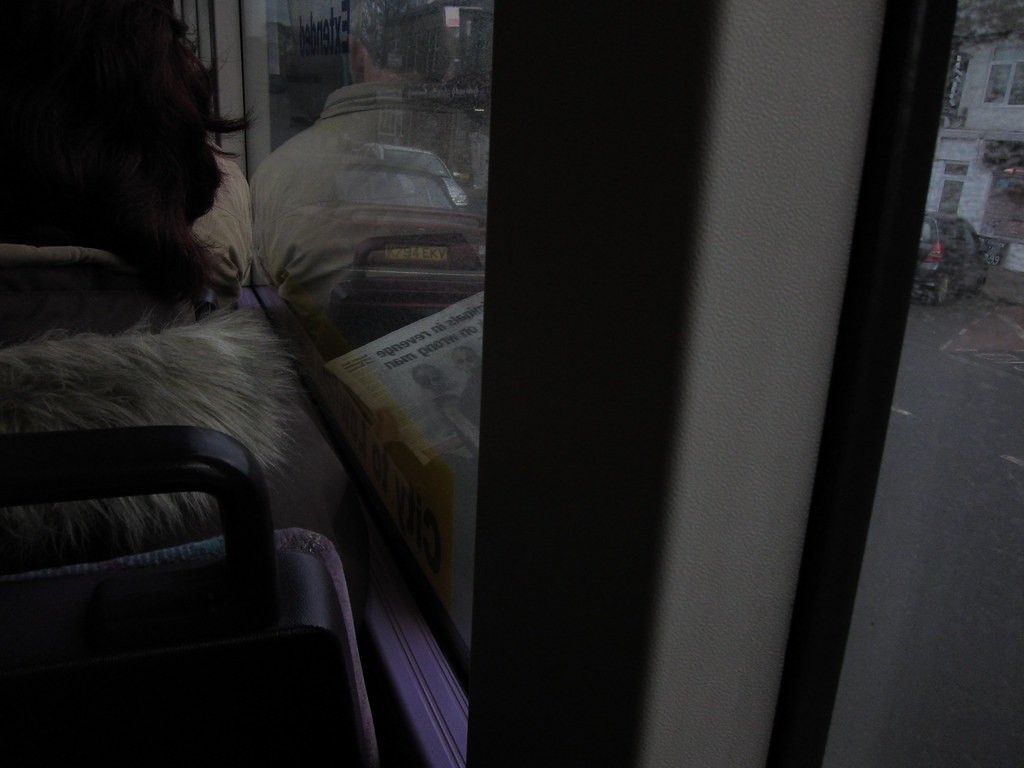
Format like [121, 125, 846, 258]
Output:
[321, 282, 486, 625]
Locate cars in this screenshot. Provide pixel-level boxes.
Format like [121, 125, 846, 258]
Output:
[298, 142, 485, 351]
[908, 210, 992, 307]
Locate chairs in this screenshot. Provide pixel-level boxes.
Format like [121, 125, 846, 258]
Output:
[0, 423, 383, 768]
[297, 205, 486, 356]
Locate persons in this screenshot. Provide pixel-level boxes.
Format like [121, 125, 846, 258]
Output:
[411, 345, 483, 457]
[246, 0, 491, 306]
[0, 0, 343, 568]
